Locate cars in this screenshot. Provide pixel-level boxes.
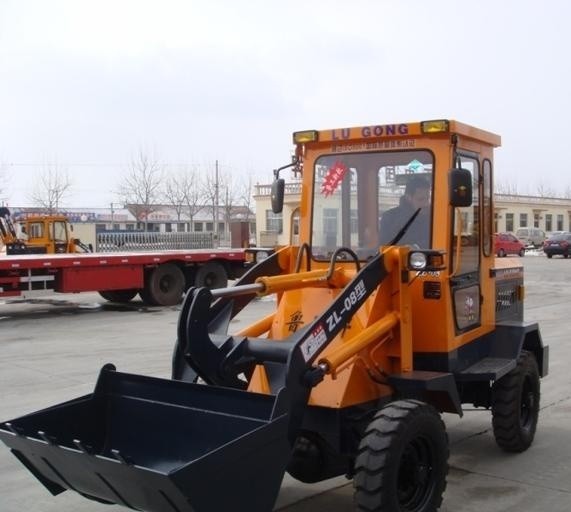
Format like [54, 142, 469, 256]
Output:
[494, 227, 571, 260]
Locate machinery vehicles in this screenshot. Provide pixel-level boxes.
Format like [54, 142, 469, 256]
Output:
[1, 208, 94, 255]
[0, 121, 548, 512]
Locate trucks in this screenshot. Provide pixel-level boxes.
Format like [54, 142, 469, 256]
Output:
[1, 248, 248, 305]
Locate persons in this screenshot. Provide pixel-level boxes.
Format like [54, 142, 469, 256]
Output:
[378, 177, 432, 250]
